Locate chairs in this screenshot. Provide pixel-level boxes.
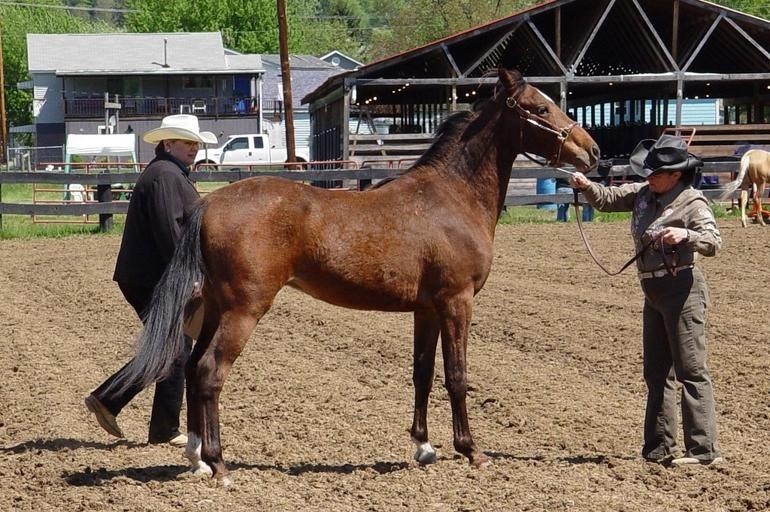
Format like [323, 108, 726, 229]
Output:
[192, 100, 206, 113]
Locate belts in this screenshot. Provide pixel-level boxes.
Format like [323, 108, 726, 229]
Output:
[637, 264, 694, 281]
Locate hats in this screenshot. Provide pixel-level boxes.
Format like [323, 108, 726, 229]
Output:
[143, 113, 219, 145]
[629, 134, 704, 178]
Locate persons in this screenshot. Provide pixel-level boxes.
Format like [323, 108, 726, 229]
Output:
[569, 133, 723, 466]
[83, 114, 207, 448]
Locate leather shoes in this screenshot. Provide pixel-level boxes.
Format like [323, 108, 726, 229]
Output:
[669, 453, 723, 467]
[148, 432, 192, 447]
[85, 394, 125, 440]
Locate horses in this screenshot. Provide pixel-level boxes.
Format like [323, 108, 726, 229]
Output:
[719, 149, 770, 228]
[123, 63, 600, 489]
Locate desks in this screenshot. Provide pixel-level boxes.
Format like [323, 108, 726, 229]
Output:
[180, 105, 191, 114]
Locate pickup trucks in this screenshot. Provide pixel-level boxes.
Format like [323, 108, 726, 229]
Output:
[190, 131, 310, 172]
